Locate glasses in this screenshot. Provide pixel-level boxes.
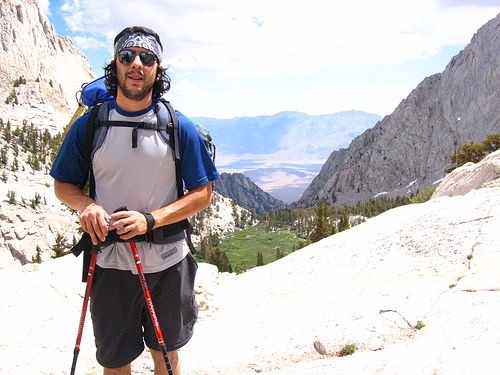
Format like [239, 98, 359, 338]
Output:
[117, 50, 155, 68]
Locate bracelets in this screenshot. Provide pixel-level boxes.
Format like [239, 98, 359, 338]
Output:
[142, 211, 154, 234]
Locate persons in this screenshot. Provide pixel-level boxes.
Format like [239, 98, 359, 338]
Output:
[51, 24, 220, 374]
